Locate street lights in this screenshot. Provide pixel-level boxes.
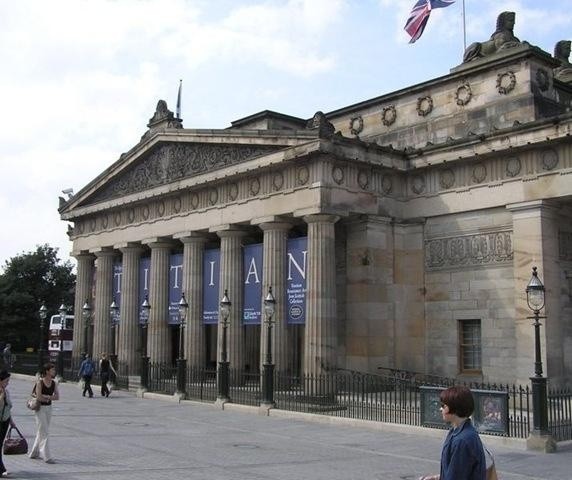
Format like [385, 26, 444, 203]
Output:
[39, 301, 48, 352]
[217, 289, 231, 399]
[141, 287, 151, 387]
[110, 295, 120, 383]
[57, 298, 67, 376]
[80, 297, 91, 361]
[260, 287, 277, 404]
[176, 293, 187, 392]
[526, 266, 553, 435]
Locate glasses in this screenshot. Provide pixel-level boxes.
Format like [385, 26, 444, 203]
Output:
[441, 402, 445, 408]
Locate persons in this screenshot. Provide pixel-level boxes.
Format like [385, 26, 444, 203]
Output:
[28, 363, 59, 464]
[2, 342, 16, 369]
[417, 385, 486, 480]
[0, 369, 16, 478]
[77, 352, 118, 398]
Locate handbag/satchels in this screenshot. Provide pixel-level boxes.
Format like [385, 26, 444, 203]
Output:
[26, 378, 43, 411]
[483, 444, 498, 480]
[3, 425, 28, 455]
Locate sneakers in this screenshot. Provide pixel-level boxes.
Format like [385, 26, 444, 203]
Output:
[82, 393, 111, 398]
[0, 455, 55, 478]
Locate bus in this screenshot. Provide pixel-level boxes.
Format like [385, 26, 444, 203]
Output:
[48, 314, 74, 363]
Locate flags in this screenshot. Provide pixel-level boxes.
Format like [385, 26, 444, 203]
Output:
[403, 0, 456, 44]
